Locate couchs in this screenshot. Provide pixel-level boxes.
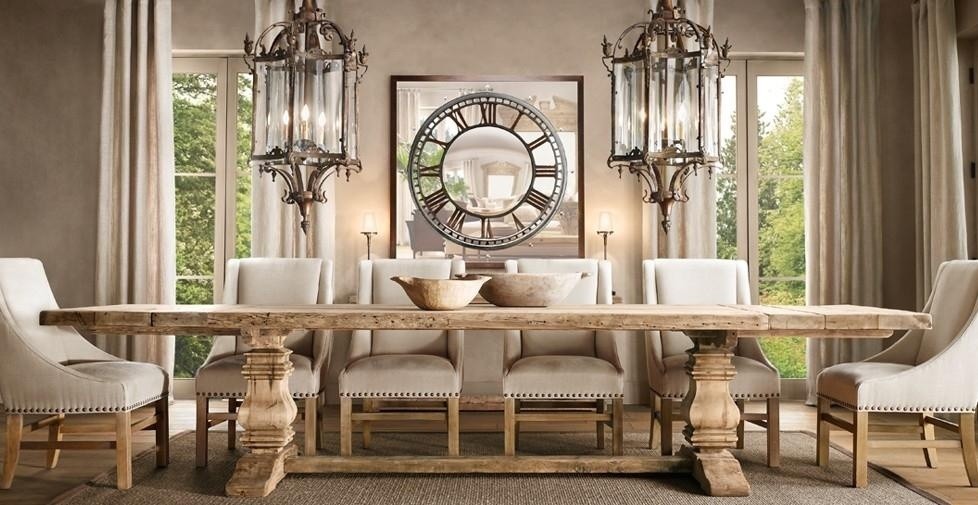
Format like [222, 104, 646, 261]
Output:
[403, 197, 580, 257]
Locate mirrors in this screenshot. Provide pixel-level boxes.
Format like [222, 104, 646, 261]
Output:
[384, 71, 588, 259]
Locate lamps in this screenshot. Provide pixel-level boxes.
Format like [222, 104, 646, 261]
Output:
[237, 1, 372, 239]
[594, 209, 615, 262]
[358, 209, 380, 258]
[599, 1, 732, 239]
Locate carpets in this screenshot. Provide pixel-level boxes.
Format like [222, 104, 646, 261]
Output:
[26, 423, 959, 505]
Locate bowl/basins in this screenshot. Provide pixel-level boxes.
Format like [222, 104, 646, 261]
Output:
[455, 272, 591, 306]
[389, 275, 493, 310]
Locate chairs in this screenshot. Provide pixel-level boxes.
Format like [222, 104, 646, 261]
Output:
[338, 259, 465, 457]
[641, 258, 781, 469]
[1, 253, 172, 497]
[503, 258, 626, 455]
[810, 256, 978, 492]
[192, 259, 334, 471]
